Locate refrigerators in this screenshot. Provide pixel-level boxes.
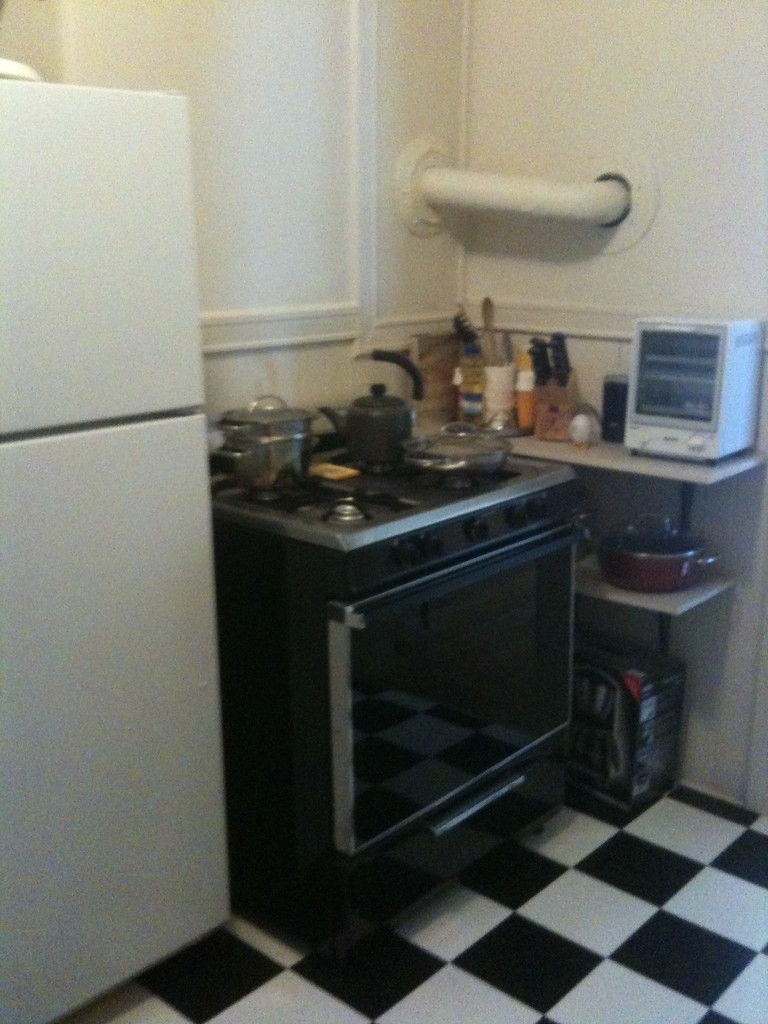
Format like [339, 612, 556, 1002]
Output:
[0, 81, 232, 1024]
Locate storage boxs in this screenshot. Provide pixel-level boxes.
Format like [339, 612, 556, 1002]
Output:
[568, 621, 687, 805]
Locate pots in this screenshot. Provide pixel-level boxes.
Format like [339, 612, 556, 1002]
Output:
[404, 421, 512, 476]
[215, 394, 314, 488]
[593, 513, 722, 594]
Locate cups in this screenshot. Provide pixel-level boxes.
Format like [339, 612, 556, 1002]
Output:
[484, 366, 517, 425]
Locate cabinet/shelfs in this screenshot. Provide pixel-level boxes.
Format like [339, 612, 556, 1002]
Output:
[507, 433, 766, 657]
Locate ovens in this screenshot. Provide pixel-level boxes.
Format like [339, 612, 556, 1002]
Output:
[210, 516, 575, 921]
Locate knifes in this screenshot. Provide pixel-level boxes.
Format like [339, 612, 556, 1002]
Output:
[528, 331, 570, 387]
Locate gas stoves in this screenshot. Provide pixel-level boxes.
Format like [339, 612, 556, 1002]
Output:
[208, 460, 579, 586]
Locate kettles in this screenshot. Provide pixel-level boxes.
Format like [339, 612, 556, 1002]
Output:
[317, 351, 423, 477]
